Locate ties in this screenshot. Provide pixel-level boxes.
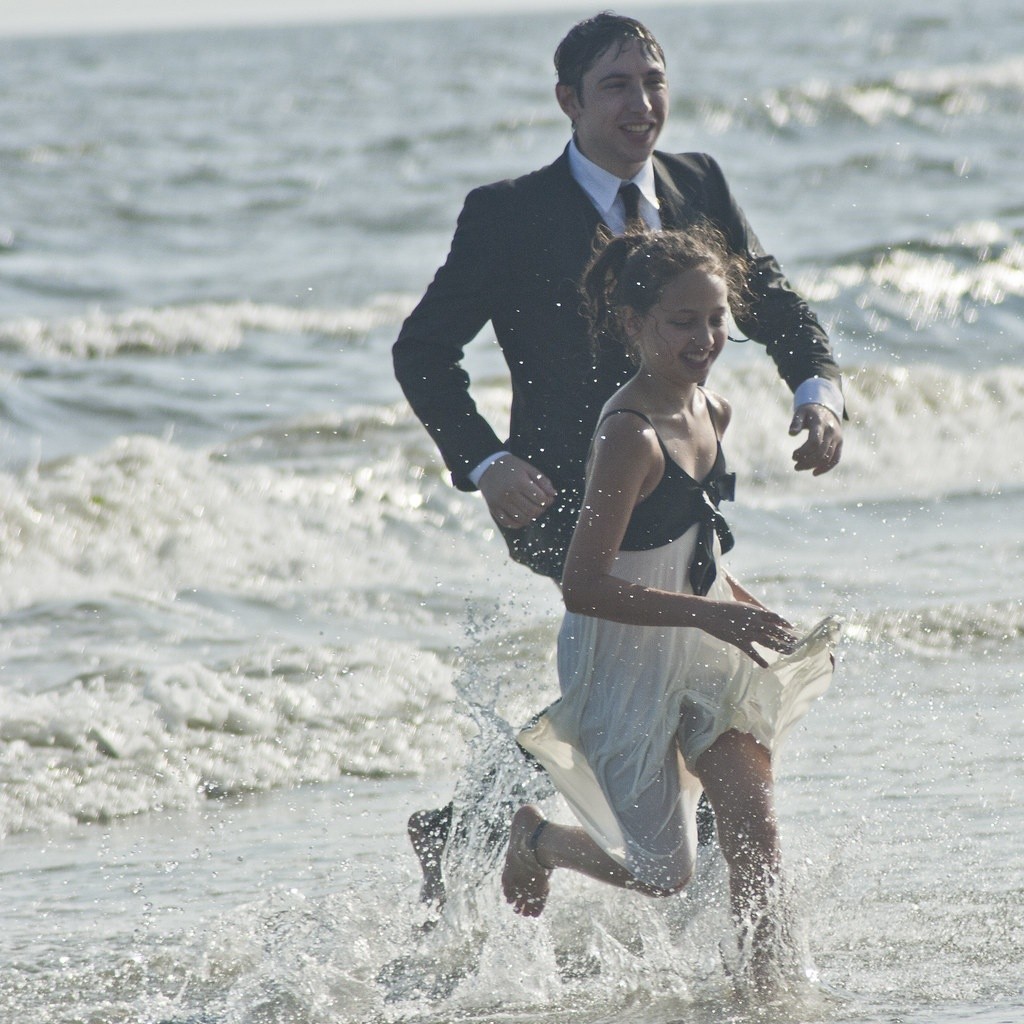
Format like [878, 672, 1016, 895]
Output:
[620, 183, 642, 229]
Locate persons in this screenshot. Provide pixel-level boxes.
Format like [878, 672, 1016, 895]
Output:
[389, 13, 849, 932]
[500, 230, 843, 984]
